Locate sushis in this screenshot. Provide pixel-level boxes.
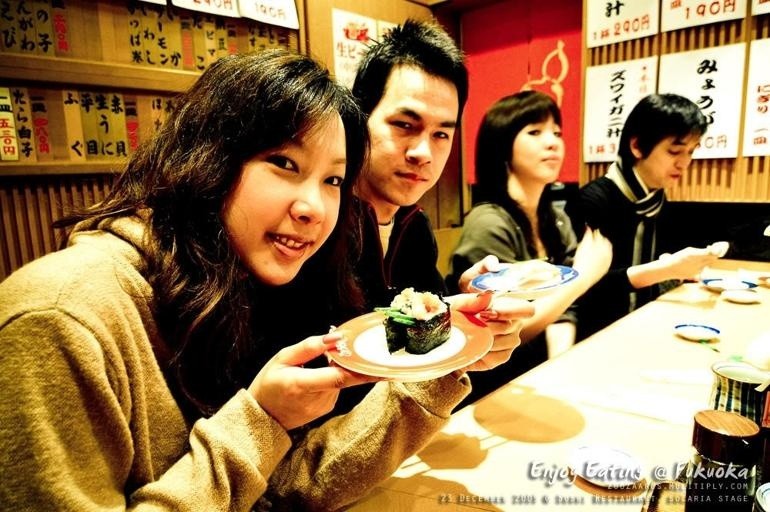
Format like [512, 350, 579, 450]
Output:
[373, 287, 451, 355]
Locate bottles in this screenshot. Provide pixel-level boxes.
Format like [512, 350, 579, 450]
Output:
[686, 361, 769, 512]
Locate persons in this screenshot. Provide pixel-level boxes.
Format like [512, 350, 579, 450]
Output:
[0, 43, 542, 512]
[558, 89, 723, 353]
[437, 87, 618, 419]
[346, 13, 530, 458]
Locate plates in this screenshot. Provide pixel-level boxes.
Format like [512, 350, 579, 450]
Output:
[565, 448, 644, 489]
[702, 276, 761, 305]
[472, 261, 578, 293]
[673, 324, 717, 345]
[326, 308, 496, 383]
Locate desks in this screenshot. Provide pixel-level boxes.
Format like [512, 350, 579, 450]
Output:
[338, 258, 770, 512]
